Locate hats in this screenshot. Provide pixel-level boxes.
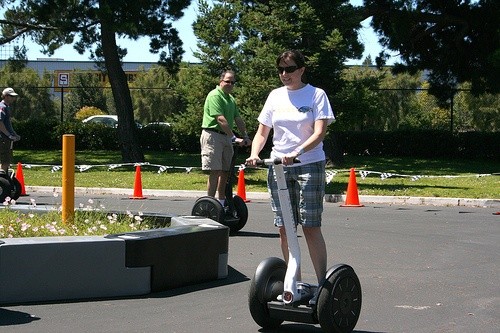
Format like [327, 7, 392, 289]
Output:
[3, 88, 18, 96]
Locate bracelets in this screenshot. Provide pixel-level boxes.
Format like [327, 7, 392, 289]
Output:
[244, 135, 249, 139]
[8, 133, 12, 138]
[295, 146, 305, 155]
[231, 135, 236, 141]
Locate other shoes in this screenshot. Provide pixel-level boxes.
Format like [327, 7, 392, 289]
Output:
[277, 279, 310, 300]
[308, 291, 319, 304]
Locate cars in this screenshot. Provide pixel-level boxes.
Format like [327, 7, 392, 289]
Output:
[83, 115, 174, 133]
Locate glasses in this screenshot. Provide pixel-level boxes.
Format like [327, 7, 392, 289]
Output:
[278, 66, 301, 73]
[222, 80, 235, 84]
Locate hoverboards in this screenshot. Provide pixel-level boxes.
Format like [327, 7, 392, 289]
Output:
[190, 140, 248, 234]
[243, 158, 363, 333]
[0, 138, 22, 203]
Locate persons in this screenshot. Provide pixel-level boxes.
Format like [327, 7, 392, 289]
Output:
[200, 71, 253, 213]
[245, 49, 336, 304]
[0, 87, 19, 182]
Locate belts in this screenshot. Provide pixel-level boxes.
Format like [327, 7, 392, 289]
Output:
[207, 127, 226, 134]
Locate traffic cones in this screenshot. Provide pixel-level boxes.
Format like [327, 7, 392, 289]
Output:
[339, 167, 365, 207]
[129, 166, 147, 199]
[15, 162, 30, 196]
[235, 170, 250, 202]
